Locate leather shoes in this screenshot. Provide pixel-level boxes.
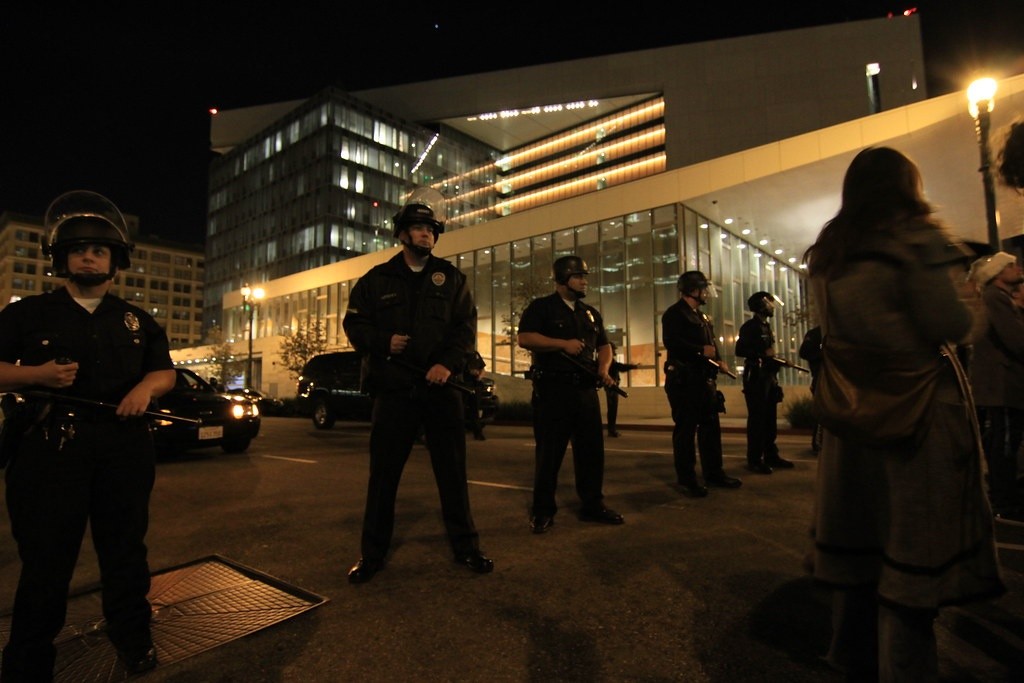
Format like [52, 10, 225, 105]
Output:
[346, 554, 381, 584]
[577, 502, 625, 525]
[529, 507, 554, 534]
[450, 547, 494, 573]
[118, 644, 159, 676]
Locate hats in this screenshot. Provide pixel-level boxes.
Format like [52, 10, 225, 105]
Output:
[977, 250, 1017, 286]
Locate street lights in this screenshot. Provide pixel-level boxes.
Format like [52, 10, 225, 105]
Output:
[966, 76, 1005, 254]
[239, 282, 267, 386]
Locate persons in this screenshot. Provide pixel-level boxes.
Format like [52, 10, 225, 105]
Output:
[663, 271, 742, 498]
[604, 341, 641, 437]
[462, 352, 486, 441]
[736, 291, 795, 474]
[800, 119, 1024, 683]
[517, 255, 625, 534]
[342, 203, 494, 586]
[0, 213, 177, 683]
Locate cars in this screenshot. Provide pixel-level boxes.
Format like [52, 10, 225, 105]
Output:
[142, 367, 262, 453]
[219, 382, 281, 413]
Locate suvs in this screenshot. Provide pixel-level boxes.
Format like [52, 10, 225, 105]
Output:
[294, 350, 500, 433]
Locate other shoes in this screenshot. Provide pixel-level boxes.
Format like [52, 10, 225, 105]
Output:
[703, 471, 743, 489]
[745, 460, 773, 474]
[677, 480, 709, 498]
[608, 431, 620, 437]
[762, 455, 795, 469]
[473, 433, 485, 440]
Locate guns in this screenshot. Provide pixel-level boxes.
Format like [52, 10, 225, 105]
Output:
[525, 369, 544, 396]
[359, 356, 369, 395]
[0, 393, 27, 469]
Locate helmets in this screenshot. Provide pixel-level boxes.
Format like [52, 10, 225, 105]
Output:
[38, 190, 129, 256]
[550, 255, 591, 279]
[747, 291, 775, 312]
[677, 271, 712, 295]
[390, 186, 447, 237]
